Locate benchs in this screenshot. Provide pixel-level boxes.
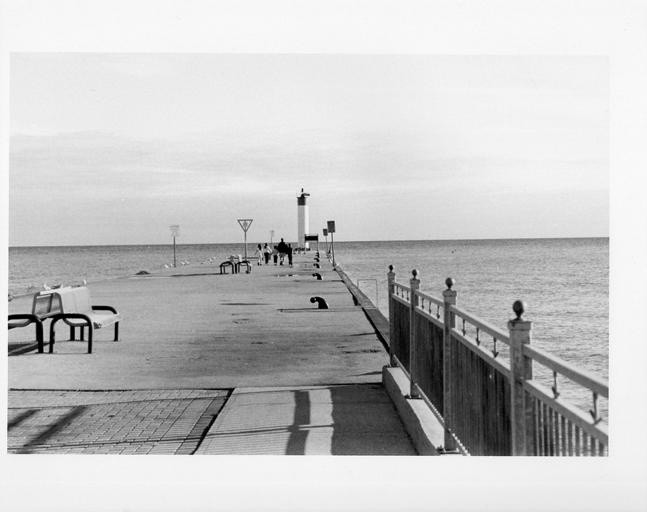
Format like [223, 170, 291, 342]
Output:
[8, 282, 121, 354]
[219, 254, 252, 274]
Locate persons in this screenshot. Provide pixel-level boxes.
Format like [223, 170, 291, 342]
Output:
[276, 237, 286, 265]
[263, 243, 272, 264]
[286, 243, 293, 268]
[253, 244, 262, 266]
[271, 245, 278, 265]
